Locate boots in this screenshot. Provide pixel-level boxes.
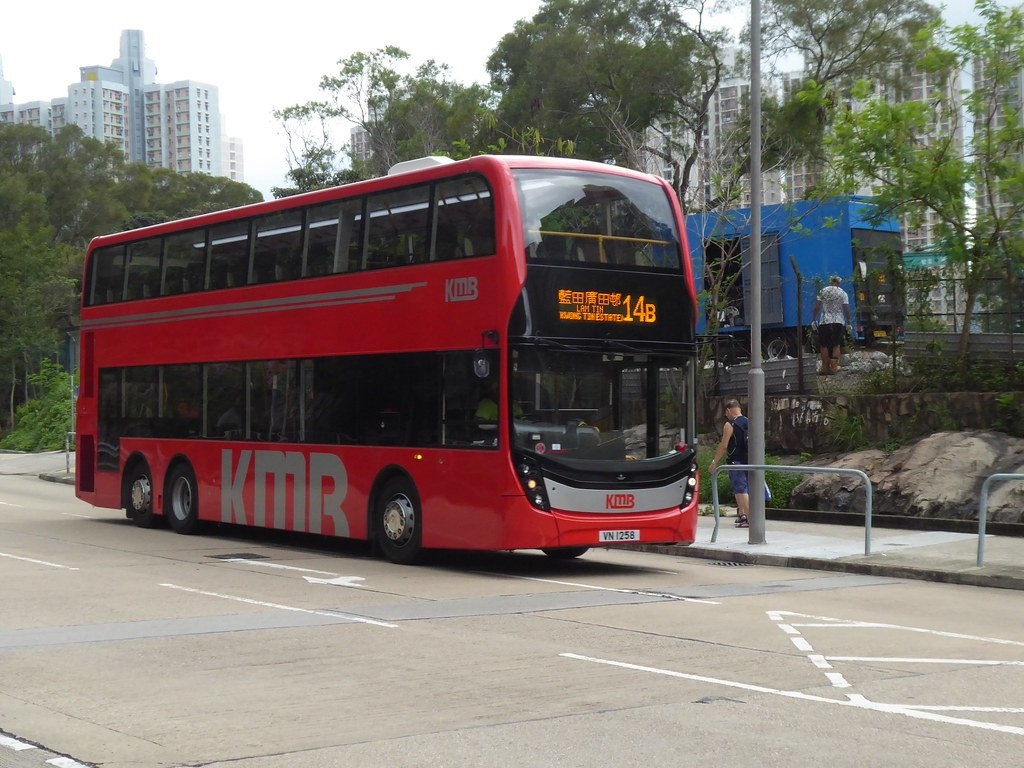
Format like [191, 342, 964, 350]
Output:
[817, 357, 837, 376]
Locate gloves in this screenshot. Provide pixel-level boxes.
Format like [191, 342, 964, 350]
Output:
[812, 320, 818, 330]
[846, 325, 853, 334]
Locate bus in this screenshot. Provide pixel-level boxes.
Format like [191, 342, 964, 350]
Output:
[69, 155, 701, 565]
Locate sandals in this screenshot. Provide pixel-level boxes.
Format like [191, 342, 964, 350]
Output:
[736, 520, 750, 527]
[735, 515, 747, 522]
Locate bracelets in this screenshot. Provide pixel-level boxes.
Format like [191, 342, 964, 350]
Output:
[712, 459, 719, 465]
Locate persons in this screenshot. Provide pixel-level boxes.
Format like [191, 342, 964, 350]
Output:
[708, 400, 749, 528]
[811, 274, 851, 374]
[139, 381, 246, 440]
[474, 379, 523, 440]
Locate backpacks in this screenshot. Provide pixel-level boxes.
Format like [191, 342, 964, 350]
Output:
[732, 420, 748, 464]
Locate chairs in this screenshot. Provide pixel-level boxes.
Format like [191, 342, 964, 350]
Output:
[569, 225, 641, 266]
[99, 235, 474, 305]
[146, 397, 176, 420]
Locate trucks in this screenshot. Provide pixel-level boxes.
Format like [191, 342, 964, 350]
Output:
[651, 195, 909, 360]
